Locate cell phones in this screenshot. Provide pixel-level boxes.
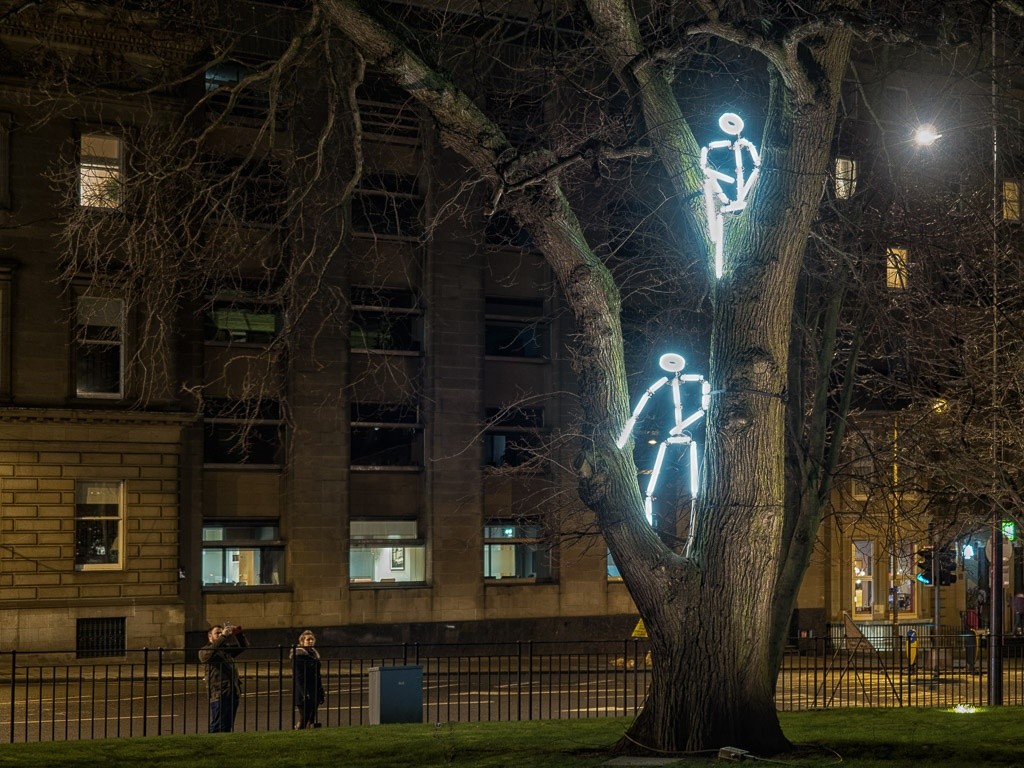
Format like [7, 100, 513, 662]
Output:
[224, 623, 231, 627]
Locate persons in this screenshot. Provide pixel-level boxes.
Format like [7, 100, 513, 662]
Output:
[197, 623, 250, 733]
[288, 630, 325, 731]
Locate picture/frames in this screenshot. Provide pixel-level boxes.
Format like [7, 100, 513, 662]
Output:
[390, 547, 405, 570]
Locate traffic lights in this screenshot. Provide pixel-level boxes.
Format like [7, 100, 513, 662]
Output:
[940, 549, 957, 586]
[916, 546, 933, 587]
[961, 543, 974, 560]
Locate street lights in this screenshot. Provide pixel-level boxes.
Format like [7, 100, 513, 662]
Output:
[915, 120, 1006, 711]
